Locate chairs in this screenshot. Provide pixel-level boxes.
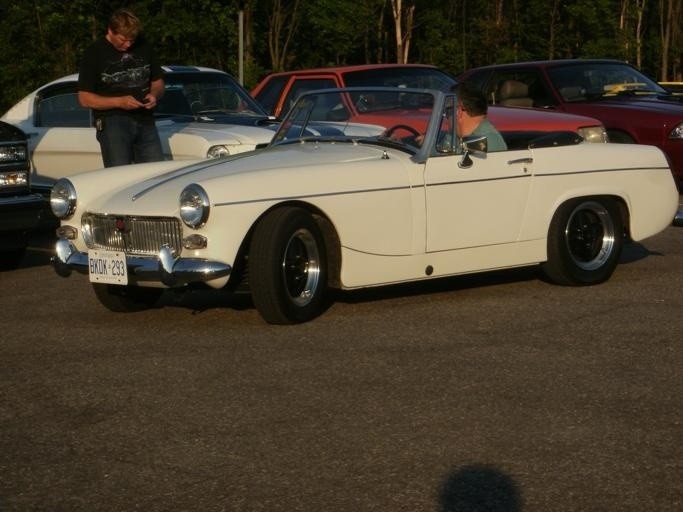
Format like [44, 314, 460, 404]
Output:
[557, 80, 584, 101]
[499, 80, 534, 107]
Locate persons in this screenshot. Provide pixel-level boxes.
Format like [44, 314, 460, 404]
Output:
[76, 9, 167, 167]
[414, 82, 509, 154]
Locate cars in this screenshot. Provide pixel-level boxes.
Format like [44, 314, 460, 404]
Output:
[48, 86, 680, 325]
